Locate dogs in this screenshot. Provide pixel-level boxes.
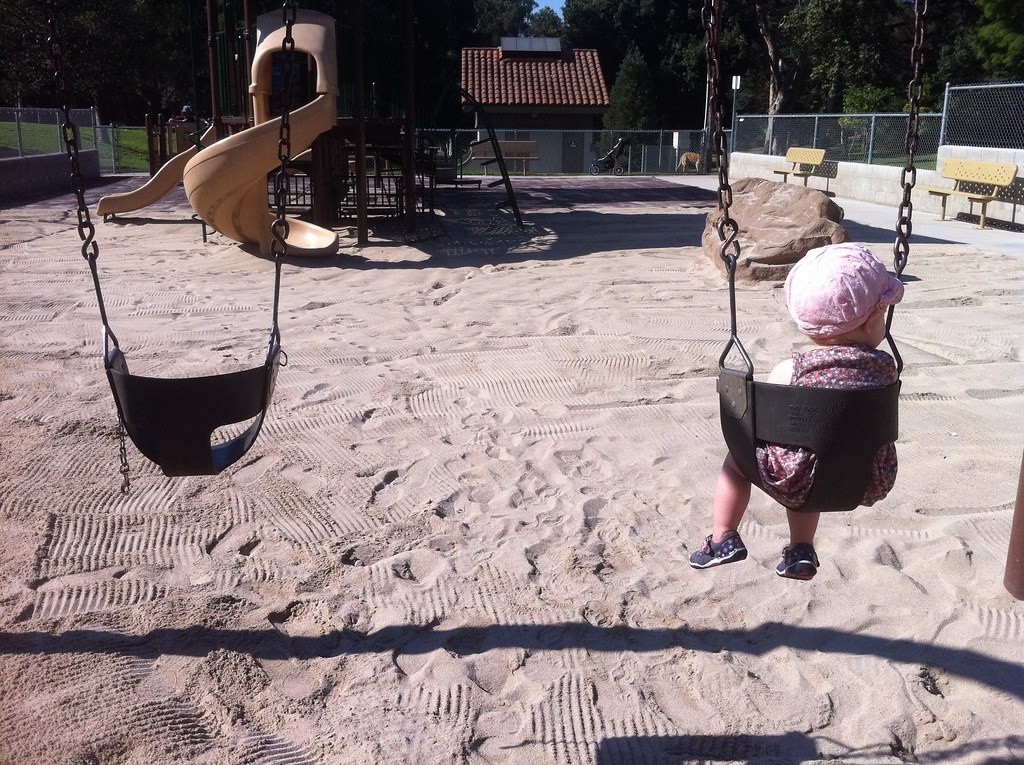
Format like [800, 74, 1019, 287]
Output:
[677, 152, 704, 172]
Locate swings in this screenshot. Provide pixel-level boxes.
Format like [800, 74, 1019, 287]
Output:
[47, 1, 298, 490]
[701, 0, 929, 511]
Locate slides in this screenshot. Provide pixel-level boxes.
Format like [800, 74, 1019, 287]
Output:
[97, 121, 215, 223]
[183, 9, 340, 258]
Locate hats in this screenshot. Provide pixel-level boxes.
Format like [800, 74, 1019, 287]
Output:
[784, 243, 906, 338]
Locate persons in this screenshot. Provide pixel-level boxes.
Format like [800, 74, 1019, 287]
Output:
[168, 106, 205, 127]
[687, 242, 906, 579]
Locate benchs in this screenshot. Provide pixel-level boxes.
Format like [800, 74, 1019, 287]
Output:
[928, 159, 1018, 230]
[775, 148, 825, 186]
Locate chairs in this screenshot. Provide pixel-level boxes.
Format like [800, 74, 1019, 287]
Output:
[470, 140, 541, 176]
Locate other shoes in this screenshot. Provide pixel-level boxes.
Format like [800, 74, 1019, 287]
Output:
[774, 546, 821, 583]
[689, 530, 748, 569]
